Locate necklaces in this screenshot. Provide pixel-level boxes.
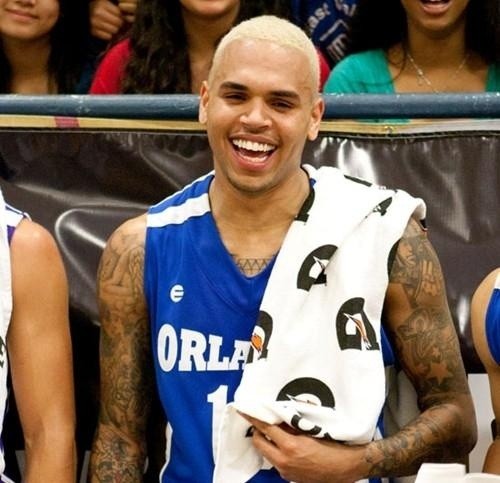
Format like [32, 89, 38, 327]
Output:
[406, 47, 473, 93]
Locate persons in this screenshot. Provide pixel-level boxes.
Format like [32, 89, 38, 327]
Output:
[88, 0, 330, 93]
[320, 0, 500, 122]
[88, 0, 136, 41]
[0, 188, 81, 482]
[0, 0, 88, 94]
[85, 14, 480, 482]
[469, 266, 499, 475]
